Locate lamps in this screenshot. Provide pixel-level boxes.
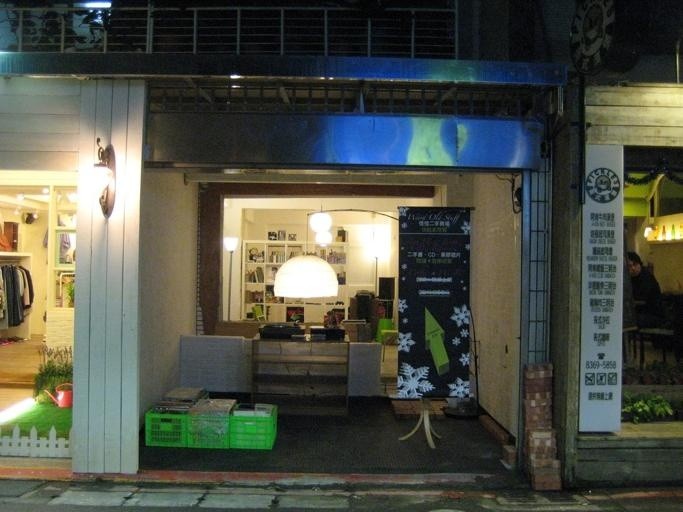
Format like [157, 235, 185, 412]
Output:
[88, 138, 116, 218]
[222, 235, 238, 321]
[272, 204, 399, 298]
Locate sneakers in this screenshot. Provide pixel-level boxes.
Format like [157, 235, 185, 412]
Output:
[0, 336, 28, 345]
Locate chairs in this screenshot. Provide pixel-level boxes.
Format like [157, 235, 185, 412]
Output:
[258, 325, 346, 341]
[618, 291, 683, 370]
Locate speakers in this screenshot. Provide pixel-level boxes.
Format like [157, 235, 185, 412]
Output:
[357, 295, 372, 319]
[379, 277, 394, 299]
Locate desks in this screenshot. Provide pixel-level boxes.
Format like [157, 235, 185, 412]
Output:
[251, 333, 349, 417]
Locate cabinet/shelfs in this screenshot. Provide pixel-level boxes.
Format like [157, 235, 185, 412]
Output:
[241, 240, 350, 322]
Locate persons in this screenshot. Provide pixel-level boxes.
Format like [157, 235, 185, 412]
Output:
[627, 250, 663, 316]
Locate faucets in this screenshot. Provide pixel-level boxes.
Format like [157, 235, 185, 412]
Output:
[294, 320, 300, 325]
[324, 323, 329, 328]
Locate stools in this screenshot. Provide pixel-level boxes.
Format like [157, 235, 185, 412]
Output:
[381, 330, 399, 362]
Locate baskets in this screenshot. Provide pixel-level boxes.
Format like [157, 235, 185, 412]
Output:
[145, 403, 278, 449]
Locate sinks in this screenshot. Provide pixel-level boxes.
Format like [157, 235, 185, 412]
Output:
[259, 324, 300, 338]
[310, 326, 345, 341]
